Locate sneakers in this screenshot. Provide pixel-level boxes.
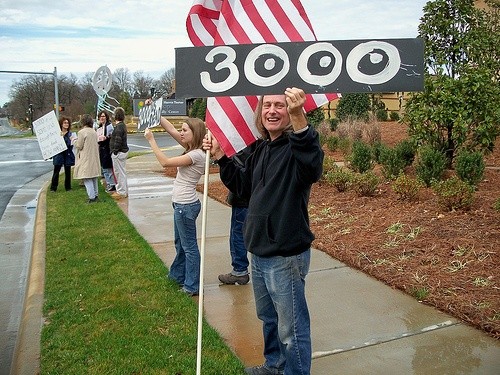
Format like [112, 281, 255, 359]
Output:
[111, 193, 128, 199]
[218, 273, 250, 285]
[246, 363, 284, 375]
[109, 185, 116, 192]
[183, 287, 204, 296]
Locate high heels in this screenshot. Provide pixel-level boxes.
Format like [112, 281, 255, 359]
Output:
[89, 196, 99, 203]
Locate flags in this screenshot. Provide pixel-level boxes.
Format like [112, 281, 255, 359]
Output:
[186, 0, 342, 159]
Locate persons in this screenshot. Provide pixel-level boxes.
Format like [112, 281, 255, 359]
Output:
[202, 87, 325, 375]
[44, 117, 75, 193]
[218, 136, 250, 285]
[144, 98, 206, 297]
[110, 107, 129, 198]
[94, 110, 116, 193]
[70, 114, 102, 204]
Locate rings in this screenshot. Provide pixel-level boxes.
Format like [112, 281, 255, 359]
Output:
[292, 99, 298, 103]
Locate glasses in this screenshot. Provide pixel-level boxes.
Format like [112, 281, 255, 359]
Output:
[100, 115, 105, 117]
